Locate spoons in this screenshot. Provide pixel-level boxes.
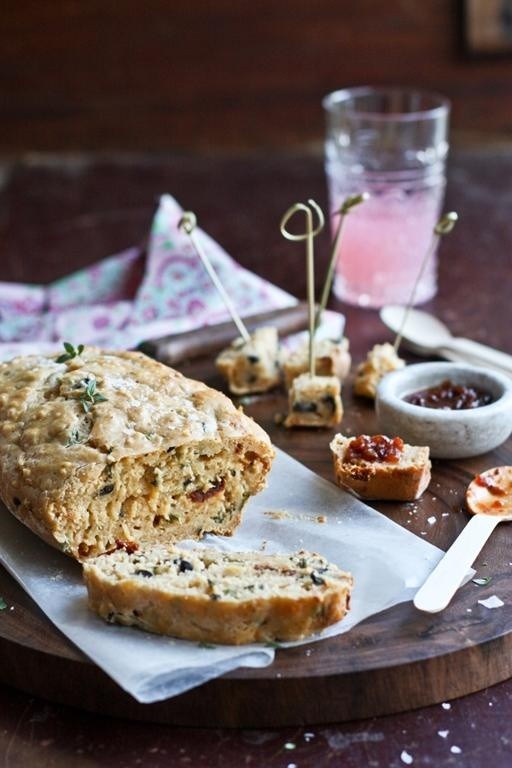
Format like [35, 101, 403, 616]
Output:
[379, 304, 512, 371]
[414, 464, 512, 616]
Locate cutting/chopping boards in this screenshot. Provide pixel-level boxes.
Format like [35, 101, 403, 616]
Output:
[1, 160, 510, 727]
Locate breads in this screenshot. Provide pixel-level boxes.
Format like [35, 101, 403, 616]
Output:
[81, 546, 353, 644]
[216, 324, 406, 428]
[331, 434, 433, 503]
[0, 343, 275, 561]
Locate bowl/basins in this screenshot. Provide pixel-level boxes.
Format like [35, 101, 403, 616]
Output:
[375, 359, 512, 460]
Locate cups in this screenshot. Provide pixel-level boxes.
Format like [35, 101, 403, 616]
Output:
[325, 83, 450, 309]
